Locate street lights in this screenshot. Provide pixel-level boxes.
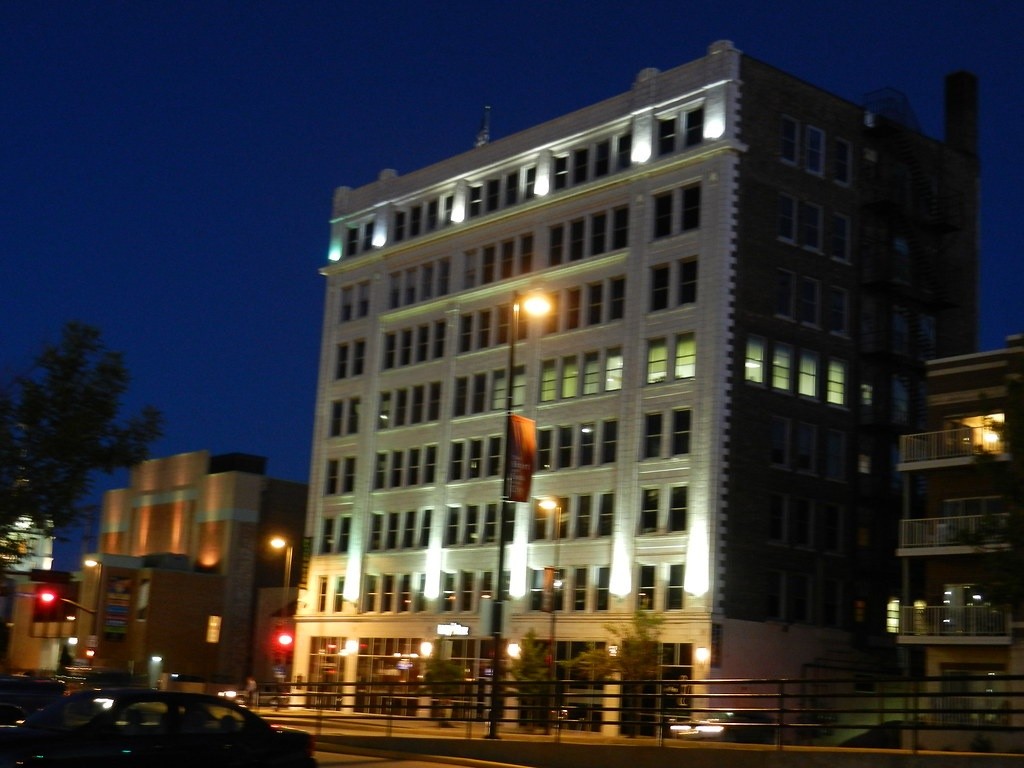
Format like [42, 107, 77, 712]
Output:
[482, 286, 552, 741]
[84, 558, 103, 665]
[269, 531, 296, 689]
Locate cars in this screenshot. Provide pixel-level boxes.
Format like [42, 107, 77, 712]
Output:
[0, 676, 66, 728]
[0, 687, 322, 768]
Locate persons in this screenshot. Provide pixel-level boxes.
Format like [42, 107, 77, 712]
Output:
[246, 676, 257, 706]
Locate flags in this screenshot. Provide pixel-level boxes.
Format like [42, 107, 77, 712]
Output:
[472, 110, 490, 149]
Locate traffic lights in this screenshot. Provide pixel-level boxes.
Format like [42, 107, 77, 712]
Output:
[41, 593, 54, 603]
[276, 630, 293, 646]
